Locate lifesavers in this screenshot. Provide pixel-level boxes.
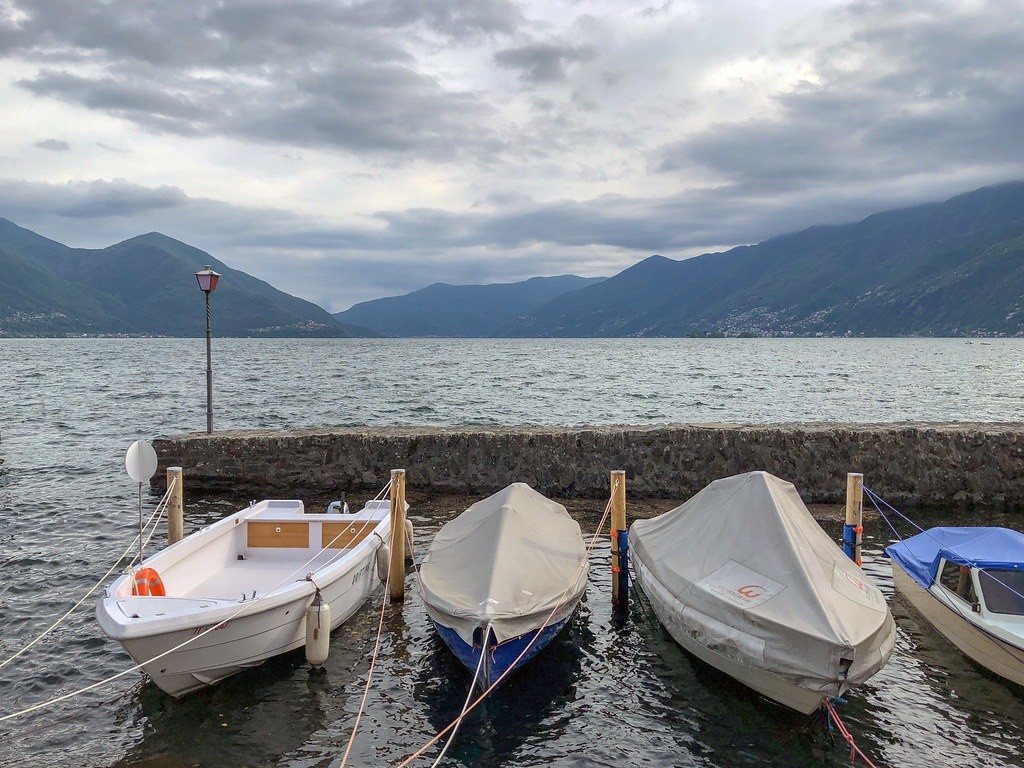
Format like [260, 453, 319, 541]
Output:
[134, 567, 167, 596]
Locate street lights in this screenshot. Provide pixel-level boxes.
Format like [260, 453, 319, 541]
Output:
[195, 264, 224, 432]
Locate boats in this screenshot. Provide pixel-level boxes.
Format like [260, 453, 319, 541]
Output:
[884, 522, 1024, 690]
[627, 467, 899, 714]
[417, 482, 590, 701]
[92, 437, 409, 703]
